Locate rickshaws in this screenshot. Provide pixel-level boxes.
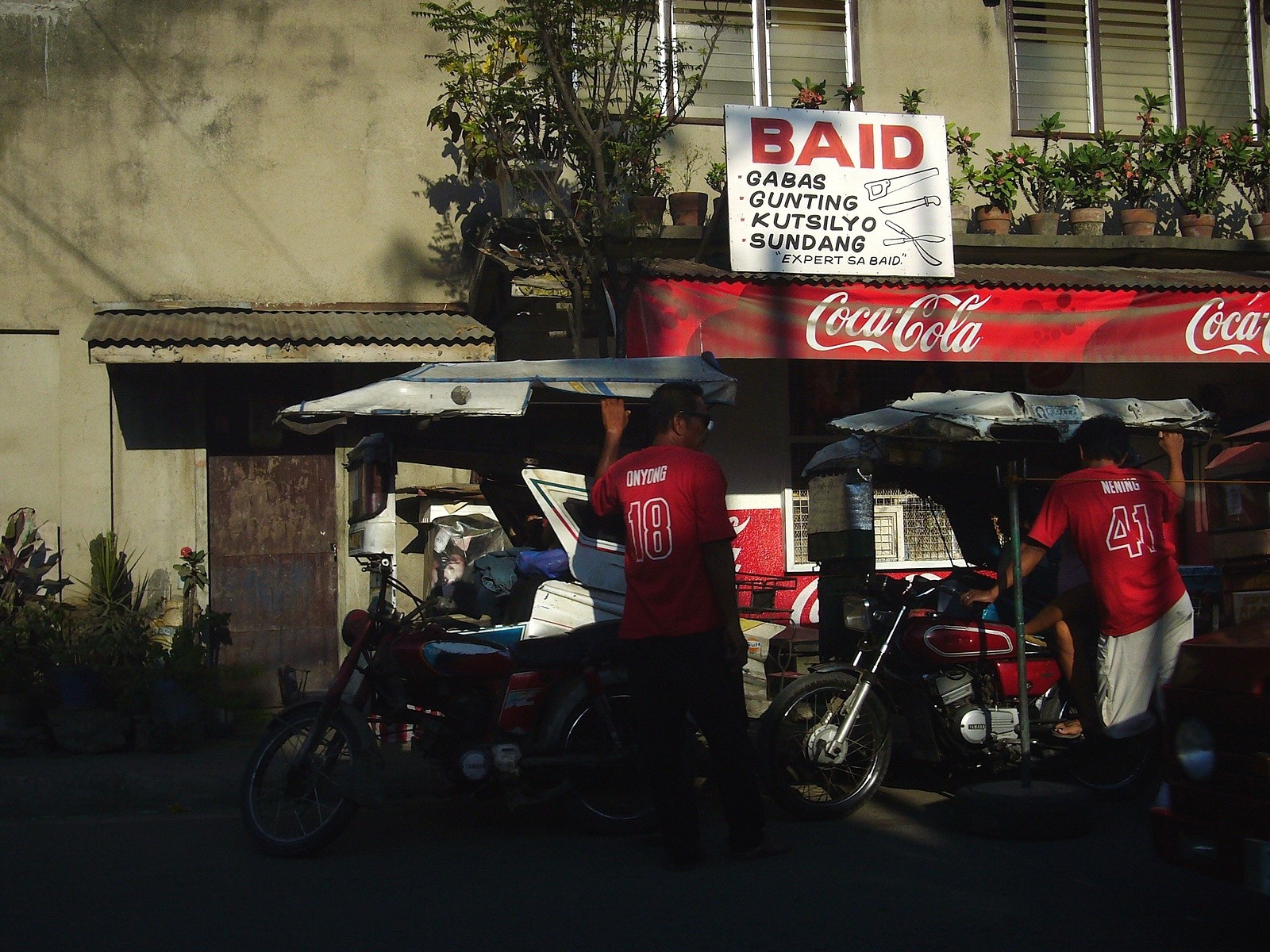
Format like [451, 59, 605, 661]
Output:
[733, 388, 1219, 837]
[245, 352, 794, 858]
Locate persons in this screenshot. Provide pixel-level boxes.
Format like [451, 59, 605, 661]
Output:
[590, 381, 787, 867]
[960, 413, 1195, 817]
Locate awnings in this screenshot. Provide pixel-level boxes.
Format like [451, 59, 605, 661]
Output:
[84, 310, 492, 363]
[622, 256, 1270, 363]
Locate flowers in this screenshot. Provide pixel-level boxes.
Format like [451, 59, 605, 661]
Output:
[624, 92, 676, 197]
[791, 77, 1270, 215]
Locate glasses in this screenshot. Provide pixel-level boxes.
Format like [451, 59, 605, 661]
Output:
[679, 409, 714, 431]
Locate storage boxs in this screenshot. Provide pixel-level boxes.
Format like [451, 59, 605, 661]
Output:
[1177, 563, 1219, 637]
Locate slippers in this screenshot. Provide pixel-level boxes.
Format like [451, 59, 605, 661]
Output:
[1052, 719, 1088, 737]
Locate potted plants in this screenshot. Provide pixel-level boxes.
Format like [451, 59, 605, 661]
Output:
[426, 25, 564, 220]
[0, 507, 172, 754]
[669, 143, 712, 226]
[704, 146, 726, 211]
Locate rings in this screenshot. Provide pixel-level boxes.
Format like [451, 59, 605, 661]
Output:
[966, 597, 969, 599]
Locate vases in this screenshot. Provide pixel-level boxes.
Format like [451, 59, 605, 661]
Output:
[1069, 208, 1106, 235]
[1028, 213, 1060, 235]
[628, 197, 666, 225]
[951, 205, 970, 234]
[1248, 213, 1270, 239]
[1178, 215, 1215, 238]
[1120, 209, 1156, 236]
[975, 205, 1013, 233]
